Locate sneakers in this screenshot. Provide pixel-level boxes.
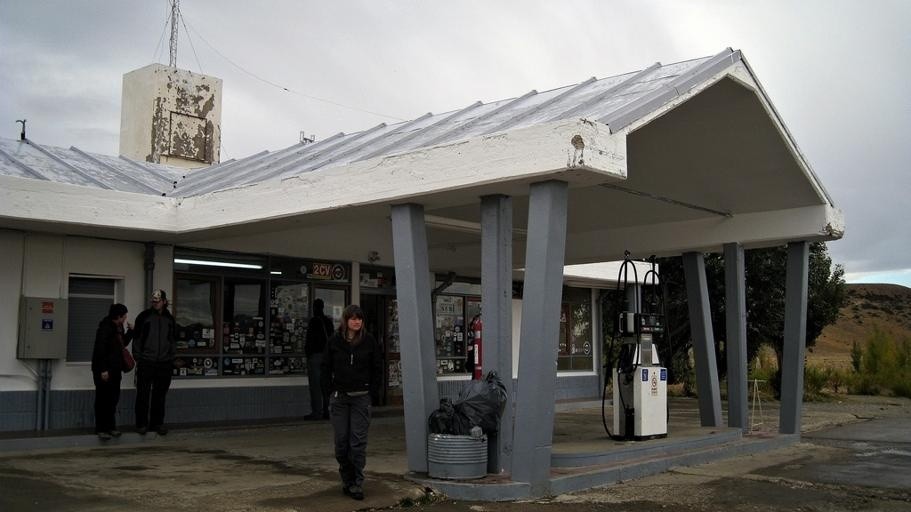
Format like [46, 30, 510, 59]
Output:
[135, 426, 147, 435]
[94, 429, 122, 440]
[342, 481, 364, 501]
[156, 427, 168, 435]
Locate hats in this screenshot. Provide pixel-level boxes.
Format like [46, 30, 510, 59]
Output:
[150, 289, 166, 303]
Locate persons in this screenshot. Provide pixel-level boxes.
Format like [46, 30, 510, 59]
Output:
[304, 299, 334, 421]
[320, 305, 380, 500]
[132, 289, 177, 434]
[92, 303, 134, 440]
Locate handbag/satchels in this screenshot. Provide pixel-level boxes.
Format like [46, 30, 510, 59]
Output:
[122, 348, 134, 373]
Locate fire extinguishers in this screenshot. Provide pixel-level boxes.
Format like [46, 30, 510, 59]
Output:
[470, 305, 482, 379]
